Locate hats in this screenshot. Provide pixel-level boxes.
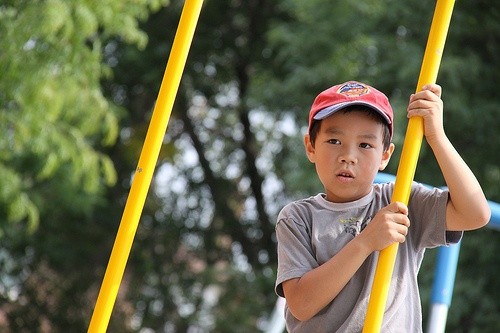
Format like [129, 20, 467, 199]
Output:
[310, 81, 394, 141]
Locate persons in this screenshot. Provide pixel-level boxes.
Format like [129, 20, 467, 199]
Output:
[273, 80, 492, 333]
